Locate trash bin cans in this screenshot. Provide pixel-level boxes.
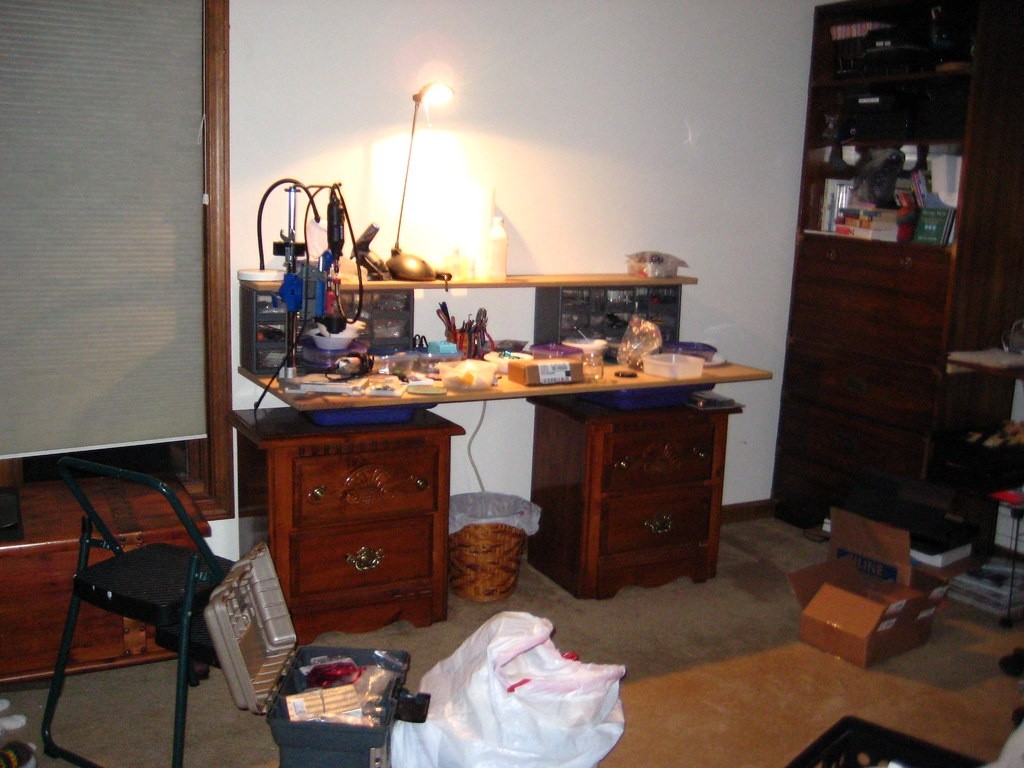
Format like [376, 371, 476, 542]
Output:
[450, 491, 536, 600]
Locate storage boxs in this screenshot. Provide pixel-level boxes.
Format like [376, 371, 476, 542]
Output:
[508, 359, 584, 384]
[0, 463, 212, 689]
[786, 507, 940, 668]
[204, 542, 431, 768]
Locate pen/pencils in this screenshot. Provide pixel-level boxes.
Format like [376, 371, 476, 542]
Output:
[435, 301, 490, 334]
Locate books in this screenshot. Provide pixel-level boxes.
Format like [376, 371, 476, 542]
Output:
[947, 348, 1024, 368]
[946, 557, 1024, 616]
[835, 170, 955, 246]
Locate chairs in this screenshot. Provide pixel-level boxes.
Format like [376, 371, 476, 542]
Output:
[42, 458, 236, 768]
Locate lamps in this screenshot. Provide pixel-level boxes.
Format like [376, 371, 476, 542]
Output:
[387, 83, 456, 280]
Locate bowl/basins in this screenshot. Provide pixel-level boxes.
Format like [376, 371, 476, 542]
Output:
[306, 329, 358, 350]
[484, 351, 534, 374]
[668, 341, 717, 361]
[641, 354, 704, 379]
[561, 338, 608, 357]
[437, 362, 496, 389]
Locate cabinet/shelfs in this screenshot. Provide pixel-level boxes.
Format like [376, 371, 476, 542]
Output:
[227, 409, 466, 649]
[526, 397, 744, 603]
[235, 268, 774, 428]
[770, 0, 1024, 544]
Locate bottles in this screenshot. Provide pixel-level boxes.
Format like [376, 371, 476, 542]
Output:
[582, 352, 604, 381]
